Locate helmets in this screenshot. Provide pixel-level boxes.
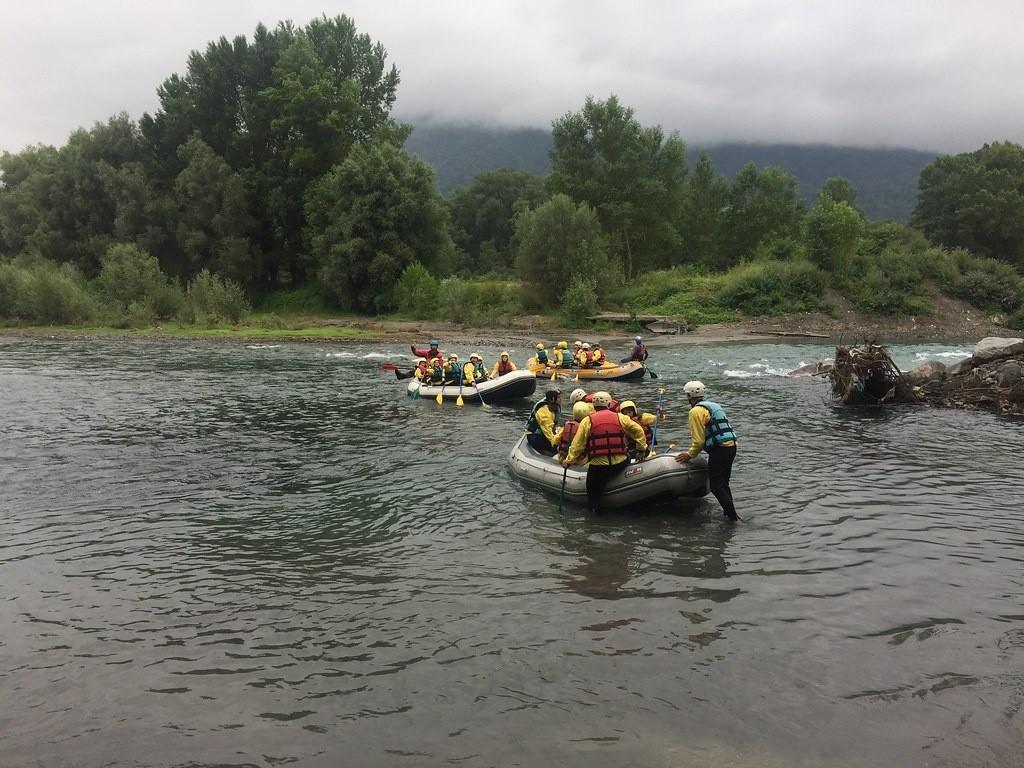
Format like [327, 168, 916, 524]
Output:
[570, 388, 588, 404]
[592, 342, 599, 348]
[537, 344, 544, 350]
[500, 352, 509, 360]
[593, 391, 612, 407]
[430, 340, 438, 350]
[558, 341, 567, 349]
[572, 402, 589, 421]
[448, 354, 458, 361]
[683, 380, 706, 398]
[574, 341, 582, 348]
[546, 387, 563, 403]
[418, 358, 426, 367]
[469, 353, 479, 360]
[478, 356, 483, 361]
[634, 336, 642, 342]
[582, 343, 589, 349]
[430, 358, 439, 368]
[620, 401, 638, 415]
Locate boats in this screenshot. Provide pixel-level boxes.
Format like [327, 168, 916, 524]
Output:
[407, 368, 537, 405]
[525, 356, 646, 380]
[508, 427, 712, 511]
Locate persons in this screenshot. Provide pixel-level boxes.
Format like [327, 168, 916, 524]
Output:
[563, 390, 648, 505]
[558, 388, 619, 465]
[395, 340, 462, 386]
[675, 380, 740, 521]
[525, 387, 565, 454]
[489, 352, 518, 379]
[619, 335, 649, 367]
[536, 343, 548, 364]
[462, 352, 489, 387]
[620, 400, 666, 460]
[552, 340, 606, 368]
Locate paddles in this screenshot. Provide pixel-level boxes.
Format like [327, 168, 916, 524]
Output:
[533, 362, 537, 379]
[382, 363, 415, 370]
[550, 366, 558, 380]
[411, 365, 429, 400]
[647, 363, 658, 379]
[456, 369, 465, 406]
[436, 382, 446, 405]
[574, 359, 581, 381]
[648, 387, 666, 457]
[475, 385, 491, 408]
[666, 444, 676, 453]
[559, 423, 576, 510]
